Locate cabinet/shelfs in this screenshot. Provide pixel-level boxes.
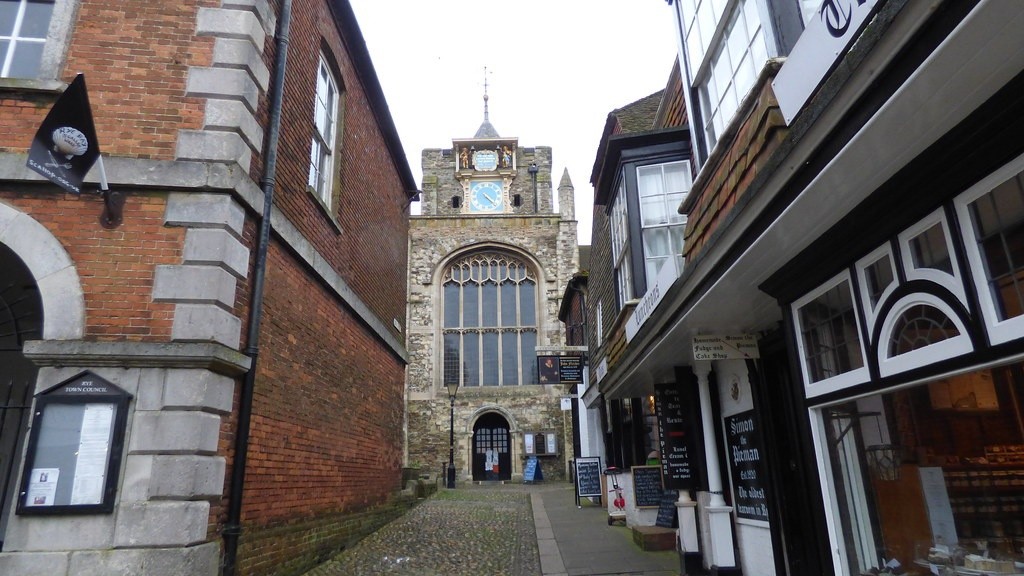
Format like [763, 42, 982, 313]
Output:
[943, 464, 1024, 557]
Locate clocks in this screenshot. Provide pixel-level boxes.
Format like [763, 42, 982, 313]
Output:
[467, 180, 504, 213]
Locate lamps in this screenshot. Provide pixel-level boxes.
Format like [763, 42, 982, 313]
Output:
[828, 411, 902, 482]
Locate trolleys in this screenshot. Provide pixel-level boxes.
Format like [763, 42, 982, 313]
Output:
[604, 467, 629, 526]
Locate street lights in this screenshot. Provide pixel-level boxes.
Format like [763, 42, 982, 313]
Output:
[445, 381, 459, 489]
